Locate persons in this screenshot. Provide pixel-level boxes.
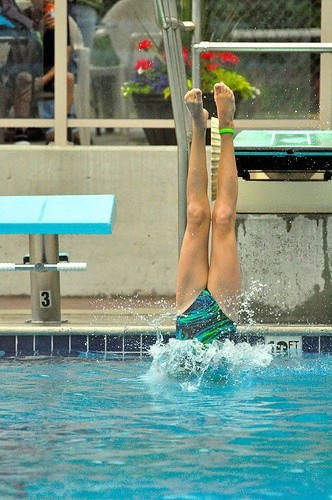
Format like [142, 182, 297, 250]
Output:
[175, 82, 242, 346]
[0, 0, 109, 147]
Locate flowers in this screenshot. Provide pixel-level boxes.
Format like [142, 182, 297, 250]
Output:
[121, 0, 261, 102]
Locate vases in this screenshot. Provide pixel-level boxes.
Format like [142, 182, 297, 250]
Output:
[131, 90, 244, 146]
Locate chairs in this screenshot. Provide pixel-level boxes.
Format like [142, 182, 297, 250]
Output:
[67, 0, 164, 145]
[0, 25, 54, 99]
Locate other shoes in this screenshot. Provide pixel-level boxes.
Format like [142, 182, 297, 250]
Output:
[14, 128, 31, 145]
